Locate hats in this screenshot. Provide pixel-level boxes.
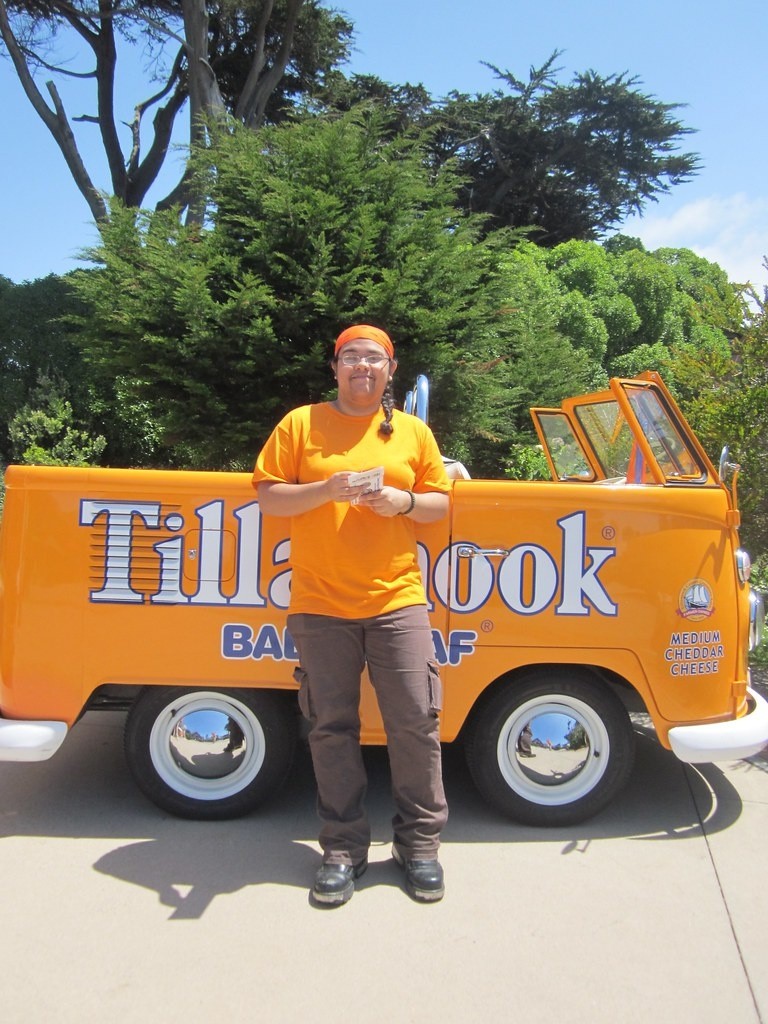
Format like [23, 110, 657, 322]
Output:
[334, 325, 394, 361]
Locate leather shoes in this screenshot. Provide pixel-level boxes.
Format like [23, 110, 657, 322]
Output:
[392, 842, 445, 902]
[313, 854, 368, 904]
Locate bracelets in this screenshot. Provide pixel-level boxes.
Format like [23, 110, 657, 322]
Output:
[397, 490, 415, 516]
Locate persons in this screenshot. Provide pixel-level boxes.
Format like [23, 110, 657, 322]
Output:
[251, 326, 455, 905]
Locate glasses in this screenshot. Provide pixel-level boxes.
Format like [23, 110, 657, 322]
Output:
[337, 354, 391, 365]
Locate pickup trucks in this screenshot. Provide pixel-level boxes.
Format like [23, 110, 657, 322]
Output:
[1, 368, 768, 829]
